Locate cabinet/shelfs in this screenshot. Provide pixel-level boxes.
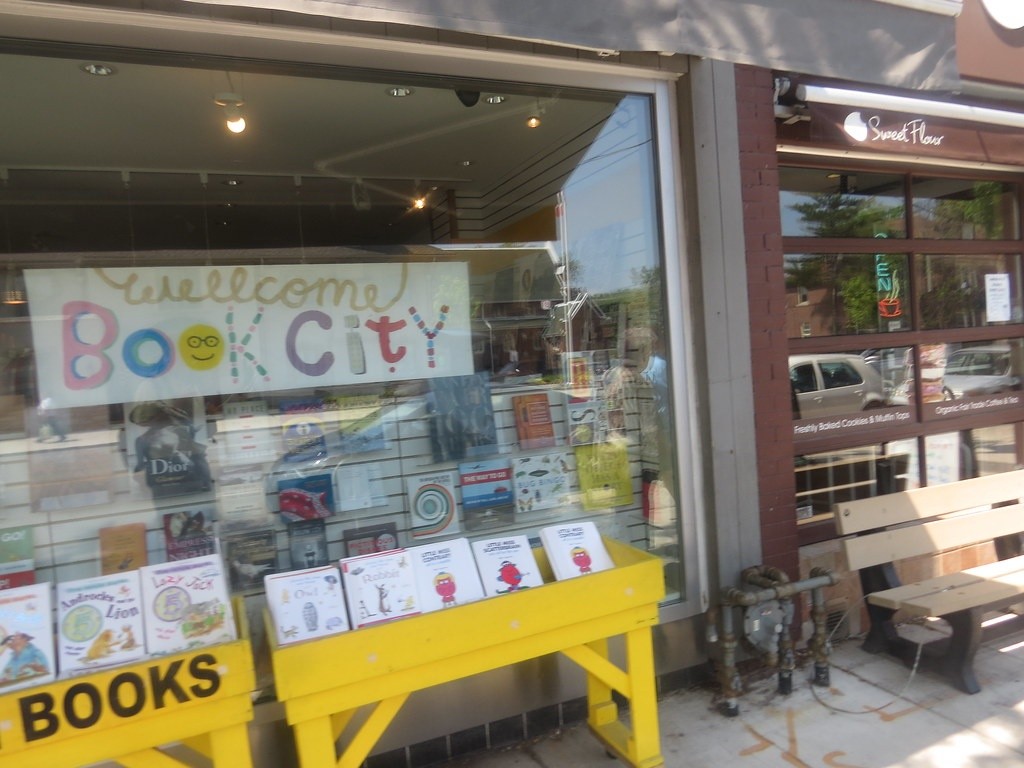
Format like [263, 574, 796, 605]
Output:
[0, 383, 647, 660]
[795, 445, 912, 525]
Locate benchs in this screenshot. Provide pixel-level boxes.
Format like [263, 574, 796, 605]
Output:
[834, 461, 1023, 697]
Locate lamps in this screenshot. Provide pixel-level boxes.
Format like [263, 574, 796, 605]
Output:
[795, 85, 1023, 128]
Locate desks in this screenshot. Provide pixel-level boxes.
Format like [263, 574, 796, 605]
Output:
[0, 594, 257, 768]
[263, 536, 664, 768]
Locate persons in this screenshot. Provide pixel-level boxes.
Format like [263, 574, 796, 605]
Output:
[602, 327, 669, 454]
[1008, 340, 1024, 390]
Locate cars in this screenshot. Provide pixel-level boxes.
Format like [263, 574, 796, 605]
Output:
[786, 341, 1020, 422]
[263, 381, 595, 523]
